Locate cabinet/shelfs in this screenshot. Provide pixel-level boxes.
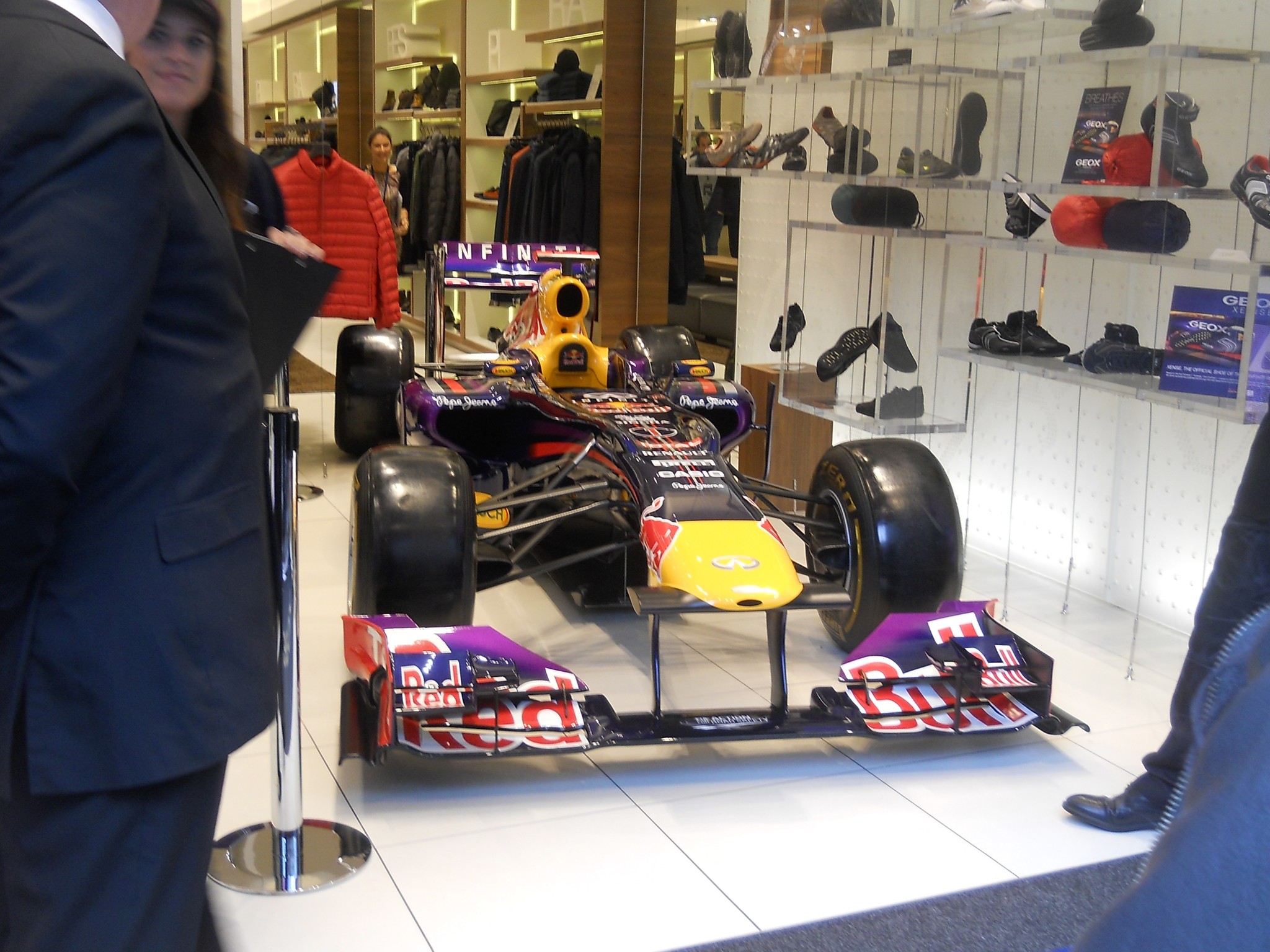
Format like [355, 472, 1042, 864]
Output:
[232, 0, 614, 335]
[736, 360, 839, 514]
[685, 0, 1270, 446]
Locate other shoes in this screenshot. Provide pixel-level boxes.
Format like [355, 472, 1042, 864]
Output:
[742, 101, 1268, 423]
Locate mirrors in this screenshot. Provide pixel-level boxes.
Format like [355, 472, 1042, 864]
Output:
[658, 0, 740, 401]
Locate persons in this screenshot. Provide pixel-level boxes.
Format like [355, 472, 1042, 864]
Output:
[0, 0, 281, 952]
[685, 131, 719, 207]
[123, 2, 323, 412]
[702, 165, 739, 288]
[1069, 604, 1269, 952]
[361, 127, 410, 274]
[1065, 406, 1270, 832]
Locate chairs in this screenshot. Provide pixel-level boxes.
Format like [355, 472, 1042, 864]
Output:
[534, 331, 636, 405]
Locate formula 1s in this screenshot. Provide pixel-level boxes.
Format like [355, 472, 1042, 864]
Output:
[331, 251, 965, 652]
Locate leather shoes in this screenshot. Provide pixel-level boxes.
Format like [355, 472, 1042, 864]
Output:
[1060, 770, 1181, 838]
